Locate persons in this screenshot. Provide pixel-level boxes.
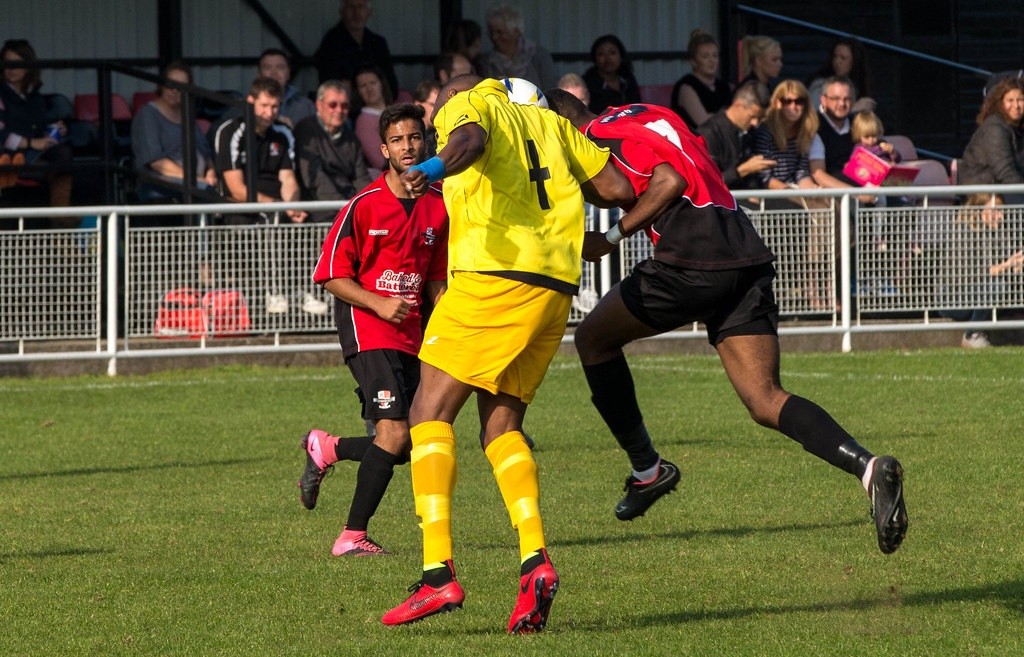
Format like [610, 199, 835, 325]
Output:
[298, 104, 448, 560]
[0, 0, 1024, 348]
[545, 88, 909, 556]
[382, 73, 639, 634]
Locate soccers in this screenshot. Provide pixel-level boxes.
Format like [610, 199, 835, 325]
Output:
[500, 77, 549, 110]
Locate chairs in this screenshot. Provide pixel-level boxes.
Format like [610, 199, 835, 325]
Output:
[15, 92, 215, 228]
[883, 135, 965, 207]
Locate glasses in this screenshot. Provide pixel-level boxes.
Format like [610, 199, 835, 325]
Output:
[784, 97, 805, 105]
[328, 100, 349, 109]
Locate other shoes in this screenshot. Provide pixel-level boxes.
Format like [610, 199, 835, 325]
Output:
[264, 295, 288, 314]
[962, 332, 993, 349]
[289, 289, 328, 315]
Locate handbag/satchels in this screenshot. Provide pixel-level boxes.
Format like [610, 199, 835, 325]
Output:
[155, 285, 252, 339]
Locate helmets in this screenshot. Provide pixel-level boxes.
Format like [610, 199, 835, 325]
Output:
[498, 77, 549, 111]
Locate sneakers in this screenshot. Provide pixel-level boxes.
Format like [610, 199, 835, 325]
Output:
[298, 429, 335, 510]
[332, 523, 394, 557]
[381, 558, 466, 625]
[506, 547, 560, 634]
[614, 459, 681, 522]
[866, 455, 909, 554]
